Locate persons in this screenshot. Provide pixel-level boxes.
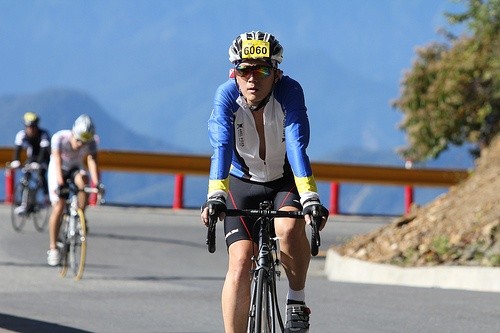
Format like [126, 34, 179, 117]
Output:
[201, 31, 329, 332]
[12, 111, 52, 214]
[47, 114, 101, 266]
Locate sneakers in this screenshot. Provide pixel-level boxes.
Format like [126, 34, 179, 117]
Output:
[284, 304, 311, 333]
[47, 249, 59, 266]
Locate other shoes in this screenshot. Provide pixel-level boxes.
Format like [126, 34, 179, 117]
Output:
[15, 206, 26, 214]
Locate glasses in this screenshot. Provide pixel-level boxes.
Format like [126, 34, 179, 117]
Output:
[234, 63, 272, 79]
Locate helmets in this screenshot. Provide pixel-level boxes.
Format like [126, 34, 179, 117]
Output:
[228, 31, 284, 65]
[24, 112, 38, 127]
[73, 114, 94, 143]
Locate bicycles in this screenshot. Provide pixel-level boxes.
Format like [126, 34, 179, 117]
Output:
[6, 160, 51, 233]
[206, 200, 322, 333]
[54, 183, 107, 286]
